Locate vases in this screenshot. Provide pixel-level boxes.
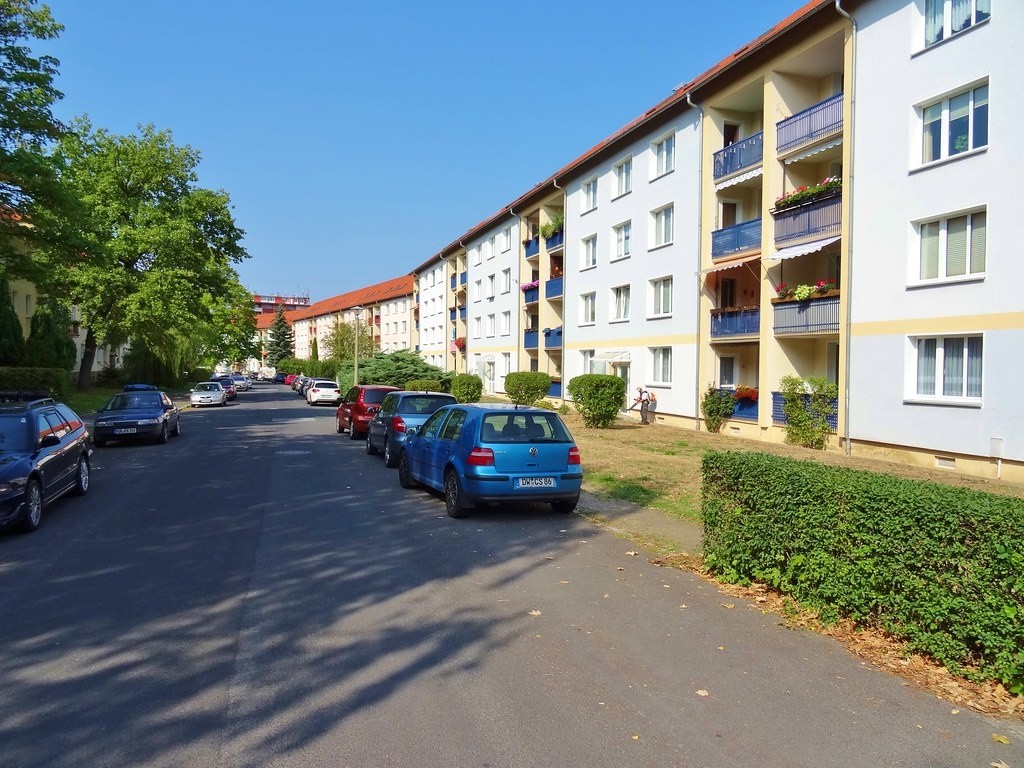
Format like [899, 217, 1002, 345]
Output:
[775, 186, 843, 214]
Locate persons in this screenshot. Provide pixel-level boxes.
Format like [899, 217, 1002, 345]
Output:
[634, 387, 650, 425]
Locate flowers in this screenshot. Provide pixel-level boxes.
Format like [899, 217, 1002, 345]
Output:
[773, 176, 841, 206]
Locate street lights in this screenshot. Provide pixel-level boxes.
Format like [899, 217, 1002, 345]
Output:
[350, 306, 366, 387]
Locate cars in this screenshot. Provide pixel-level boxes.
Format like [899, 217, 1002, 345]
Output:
[398, 403, 583, 519]
[274, 367, 342, 407]
[210, 375, 252, 401]
[365, 391, 460, 468]
[0, 398, 94, 532]
[189, 382, 227, 407]
[336, 385, 407, 439]
[93, 384, 180, 448]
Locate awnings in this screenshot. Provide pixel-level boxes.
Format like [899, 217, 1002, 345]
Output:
[695, 254, 761, 300]
[409, 303, 419, 310]
[760, 236, 841, 288]
